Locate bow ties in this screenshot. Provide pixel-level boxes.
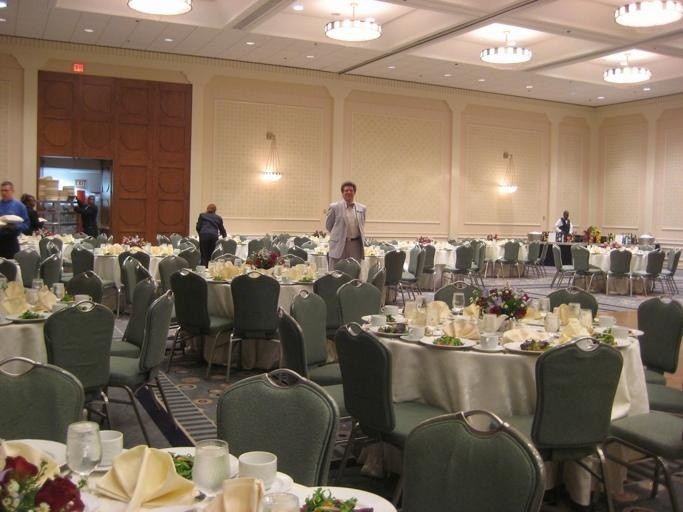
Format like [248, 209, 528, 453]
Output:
[347, 202, 354, 208]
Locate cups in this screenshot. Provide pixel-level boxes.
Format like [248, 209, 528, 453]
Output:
[381, 305, 399, 317]
[480, 333, 500, 348]
[30, 278, 43, 291]
[368, 315, 387, 327]
[53, 283, 64, 298]
[27, 289, 38, 304]
[95, 429, 123, 466]
[262, 493, 298, 512]
[74, 295, 92, 303]
[239, 449, 278, 491]
[408, 326, 426, 339]
[538, 298, 644, 350]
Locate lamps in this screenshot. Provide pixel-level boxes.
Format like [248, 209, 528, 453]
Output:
[126, 0, 192, 17]
[603, 53, 652, 84]
[260, 131, 282, 181]
[614, 0, 683, 28]
[500, 152, 518, 195]
[324, 3, 383, 41]
[480, 31, 532, 64]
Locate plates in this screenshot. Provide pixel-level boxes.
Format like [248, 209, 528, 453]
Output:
[399, 335, 428, 343]
[3, 312, 53, 323]
[156, 447, 239, 478]
[360, 314, 406, 324]
[3, 438, 67, 471]
[287, 485, 397, 512]
[503, 327, 555, 342]
[261, 471, 296, 496]
[369, 325, 415, 337]
[503, 341, 561, 355]
[93, 448, 131, 471]
[418, 336, 477, 350]
[471, 343, 505, 353]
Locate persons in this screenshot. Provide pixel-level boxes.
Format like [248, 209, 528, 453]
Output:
[19, 190, 45, 232]
[71, 194, 100, 238]
[323, 181, 367, 276]
[557, 211, 575, 239]
[0, 180, 31, 255]
[197, 203, 227, 268]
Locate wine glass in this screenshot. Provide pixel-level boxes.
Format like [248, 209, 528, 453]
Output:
[192, 438, 231, 499]
[452, 293, 465, 318]
[482, 314, 497, 335]
[67, 420, 103, 507]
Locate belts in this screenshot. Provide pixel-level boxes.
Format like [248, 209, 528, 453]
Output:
[351, 235, 360, 240]
